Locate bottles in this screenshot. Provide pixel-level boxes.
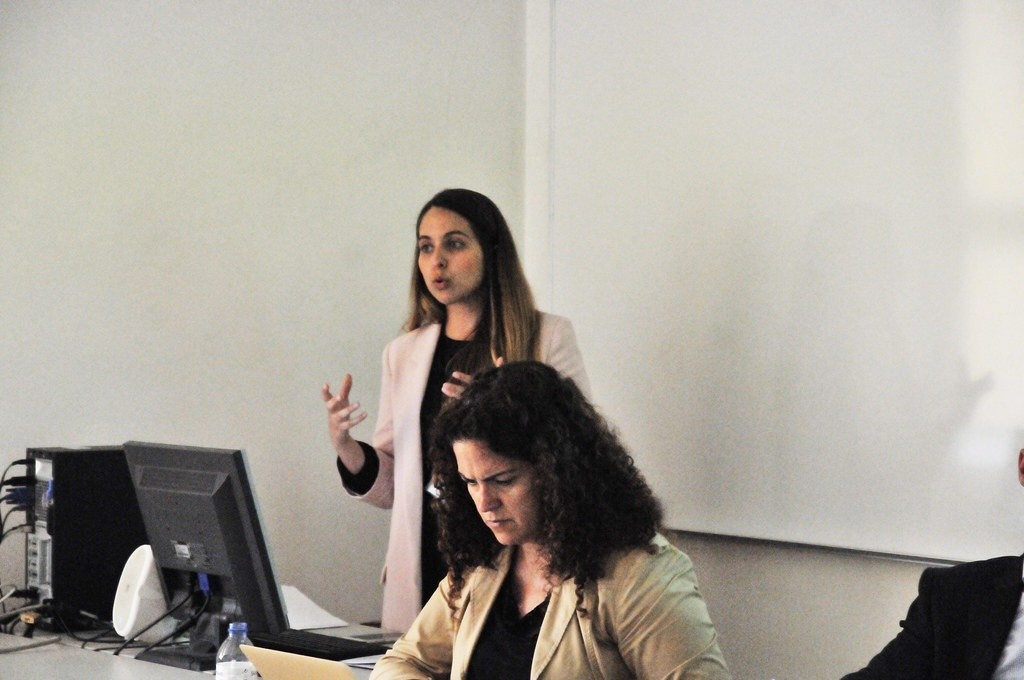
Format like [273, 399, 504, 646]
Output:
[215, 622, 258, 680]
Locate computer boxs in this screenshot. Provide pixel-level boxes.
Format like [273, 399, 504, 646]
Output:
[22, 446, 150, 632]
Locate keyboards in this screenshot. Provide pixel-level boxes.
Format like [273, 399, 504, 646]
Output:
[245, 628, 392, 663]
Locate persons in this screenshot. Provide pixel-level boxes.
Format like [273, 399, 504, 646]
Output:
[369, 360, 732, 680]
[324, 189, 592, 636]
[838, 449, 1024, 680]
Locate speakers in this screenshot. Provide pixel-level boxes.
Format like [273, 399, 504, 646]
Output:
[112, 544, 191, 645]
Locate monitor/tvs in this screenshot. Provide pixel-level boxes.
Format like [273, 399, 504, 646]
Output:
[121, 441, 290, 672]
